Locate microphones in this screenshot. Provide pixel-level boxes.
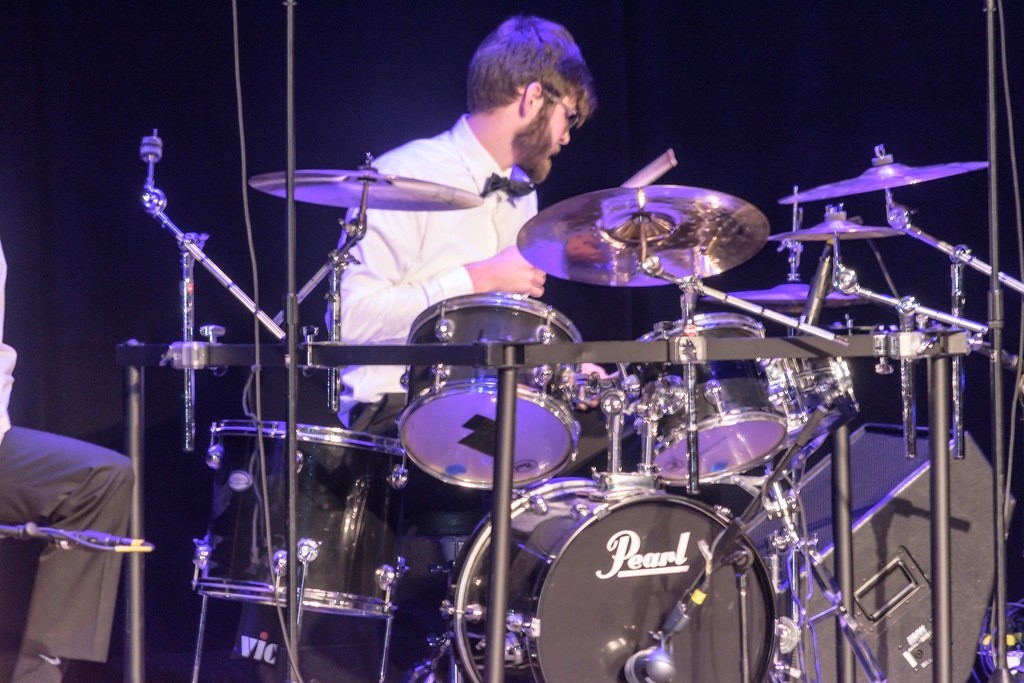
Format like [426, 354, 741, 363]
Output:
[54, 530, 156, 552]
[624, 647, 676, 683]
[800, 240, 836, 328]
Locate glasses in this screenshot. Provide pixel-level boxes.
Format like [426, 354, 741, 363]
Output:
[541, 86, 581, 130]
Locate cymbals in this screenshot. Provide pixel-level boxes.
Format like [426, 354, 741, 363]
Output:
[765, 218, 906, 243]
[699, 282, 865, 305]
[516, 182, 772, 288]
[247, 167, 485, 212]
[779, 162, 991, 207]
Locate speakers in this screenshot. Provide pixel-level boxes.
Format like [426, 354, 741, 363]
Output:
[741, 422, 1016, 683]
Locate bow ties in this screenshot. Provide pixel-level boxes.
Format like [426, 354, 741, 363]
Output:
[481, 172, 535, 198]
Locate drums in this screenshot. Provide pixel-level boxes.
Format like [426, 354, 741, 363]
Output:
[443, 473, 778, 683]
[620, 312, 790, 485]
[396, 294, 585, 490]
[757, 356, 860, 441]
[196, 421, 406, 621]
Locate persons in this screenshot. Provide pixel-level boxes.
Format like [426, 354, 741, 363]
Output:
[326, 15, 644, 608]
[1, 244, 135, 683]
